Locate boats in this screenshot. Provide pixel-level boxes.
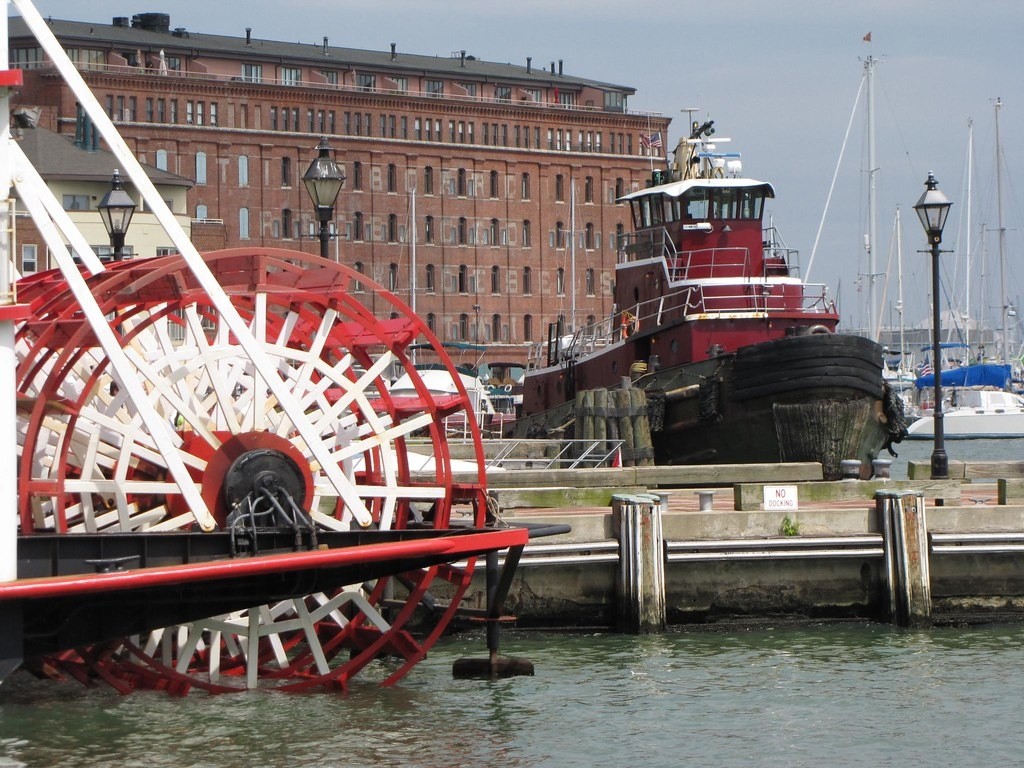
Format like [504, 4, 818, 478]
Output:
[390, 343, 516, 437]
[517, 108, 906, 482]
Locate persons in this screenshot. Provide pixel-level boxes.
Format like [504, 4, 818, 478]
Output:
[149, 64, 153, 74]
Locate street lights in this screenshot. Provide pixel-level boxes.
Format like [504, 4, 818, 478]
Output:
[301, 136, 348, 258]
[95, 168, 140, 261]
[912, 172, 957, 478]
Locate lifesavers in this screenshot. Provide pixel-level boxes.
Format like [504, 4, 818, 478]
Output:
[621, 315, 639, 338]
[487, 385, 495, 391]
[808, 323, 831, 334]
[504, 384, 512, 393]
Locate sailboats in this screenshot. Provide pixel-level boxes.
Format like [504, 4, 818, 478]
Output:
[785, 30, 1024, 440]
[341, 186, 484, 413]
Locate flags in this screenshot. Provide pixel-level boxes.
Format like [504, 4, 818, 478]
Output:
[920, 354, 931, 377]
[863, 32, 871, 42]
[642, 133, 663, 148]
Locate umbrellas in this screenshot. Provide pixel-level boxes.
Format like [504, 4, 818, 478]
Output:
[351, 69, 356, 91]
[158, 50, 168, 76]
[136, 49, 141, 64]
[554, 85, 559, 103]
